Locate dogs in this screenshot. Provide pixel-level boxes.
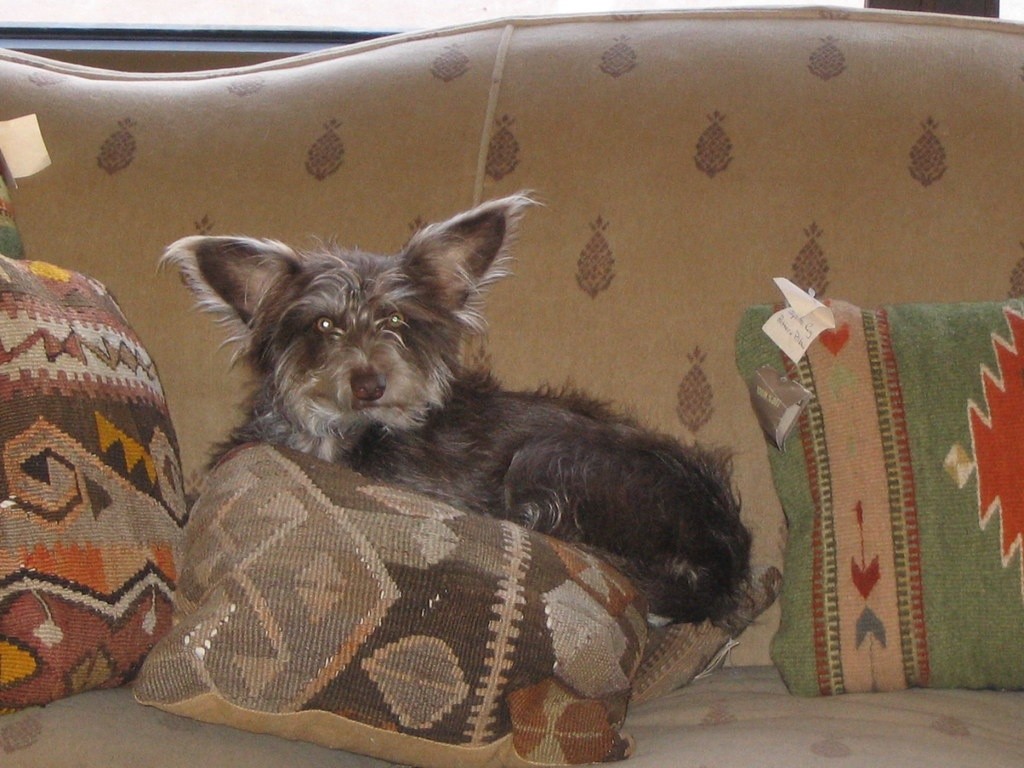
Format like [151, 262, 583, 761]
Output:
[147, 188, 757, 636]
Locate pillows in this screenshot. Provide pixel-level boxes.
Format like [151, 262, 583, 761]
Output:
[736, 300, 1024, 700]
[0, 251, 190, 719]
[128, 441, 782, 768]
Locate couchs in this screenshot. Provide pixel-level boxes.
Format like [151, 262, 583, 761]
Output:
[0, 7, 1024, 768]
